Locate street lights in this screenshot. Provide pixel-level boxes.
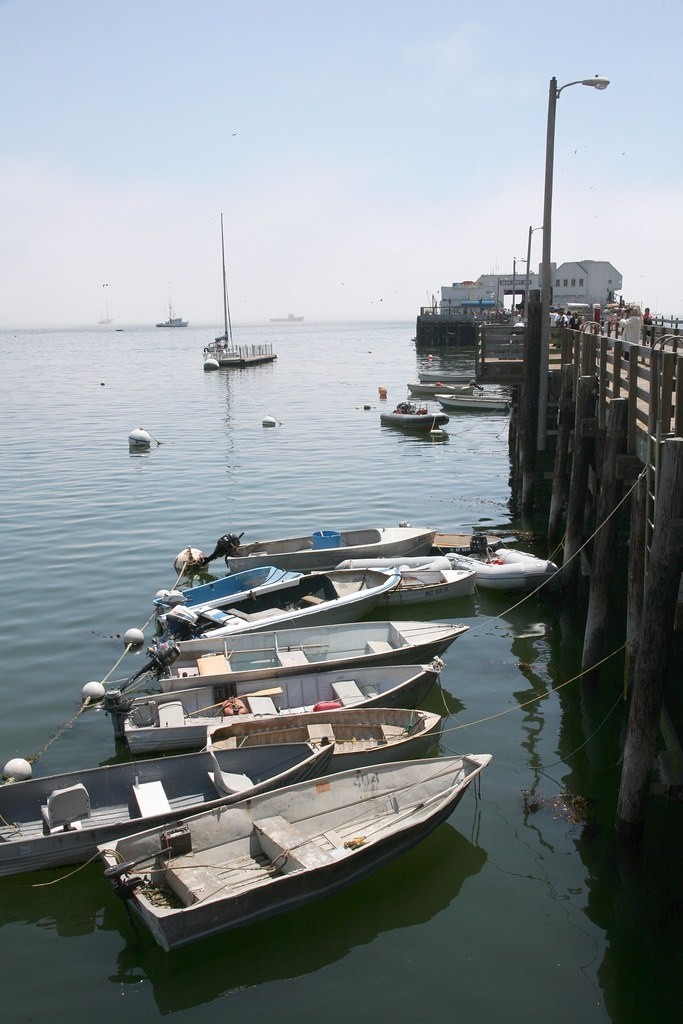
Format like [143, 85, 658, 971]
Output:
[525, 225, 544, 321]
[536, 73, 611, 449]
[512, 256, 528, 326]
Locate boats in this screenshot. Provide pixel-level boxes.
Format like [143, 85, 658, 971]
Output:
[334, 556, 453, 577]
[154, 294, 189, 328]
[407, 380, 486, 399]
[94, 752, 493, 949]
[415, 353, 477, 384]
[200, 709, 444, 767]
[398, 520, 502, 553]
[446, 531, 561, 595]
[0, 739, 338, 880]
[183, 525, 437, 575]
[111, 564, 471, 755]
[305, 568, 477, 608]
[88, 818, 489, 1020]
[376, 401, 450, 430]
[434, 392, 512, 410]
[271, 313, 304, 323]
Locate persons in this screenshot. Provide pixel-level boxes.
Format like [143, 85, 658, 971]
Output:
[643, 307, 653, 344]
[619, 305, 640, 361]
[514, 308, 524, 319]
[549, 307, 583, 348]
[579, 308, 587, 313]
[601, 307, 610, 333]
[499, 309, 511, 315]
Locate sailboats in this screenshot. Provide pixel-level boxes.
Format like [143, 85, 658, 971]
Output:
[201, 215, 277, 366]
